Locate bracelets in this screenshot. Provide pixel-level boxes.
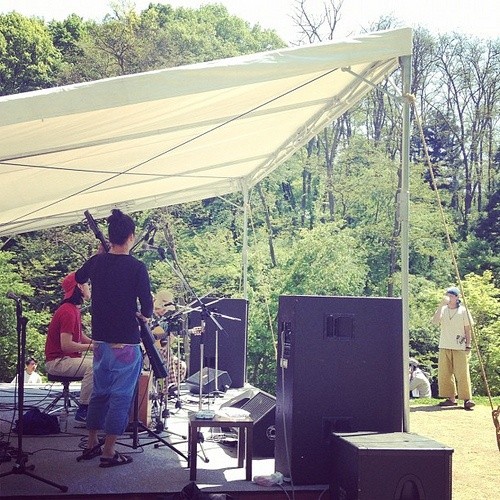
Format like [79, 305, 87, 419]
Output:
[465, 346, 472, 350]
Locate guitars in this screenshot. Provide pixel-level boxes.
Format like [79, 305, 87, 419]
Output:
[141, 325, 203, 355]
[82, 210, 168, 382]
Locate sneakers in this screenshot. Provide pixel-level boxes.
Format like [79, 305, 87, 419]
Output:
[73, 408, 87, 425]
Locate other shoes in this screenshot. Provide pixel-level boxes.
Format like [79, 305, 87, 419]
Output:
[437, 398, 476, 408]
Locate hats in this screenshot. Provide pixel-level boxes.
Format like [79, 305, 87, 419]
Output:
[63, 272, 79, 299]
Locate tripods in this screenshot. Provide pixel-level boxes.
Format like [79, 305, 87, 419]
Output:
[131, 257, 243, 463]
[1, 303, 70, 492]
[76, 346, 189, 467]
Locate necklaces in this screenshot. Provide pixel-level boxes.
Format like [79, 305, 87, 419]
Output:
[447, 305, 458, 320]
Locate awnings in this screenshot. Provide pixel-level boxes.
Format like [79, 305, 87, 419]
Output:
[1, 25, 416, 432]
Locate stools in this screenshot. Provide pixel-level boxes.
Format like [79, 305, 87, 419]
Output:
[187, 410, 253, 482]
[41, 372, 83, 433]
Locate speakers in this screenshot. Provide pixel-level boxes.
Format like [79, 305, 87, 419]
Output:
[186, 366, 232, 395]
[228, 390, 277, 459]
[187, 296, 249, 388]
[273, 294, 454, 499]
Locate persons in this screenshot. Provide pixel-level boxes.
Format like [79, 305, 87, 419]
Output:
[74, 208, 154, 469]
[407, 356, 432, 399]
[9, 358, 43, 384]
[143, 289, 186, 401]
[46, 272, 98, 429]
[430, 286, 475, 408]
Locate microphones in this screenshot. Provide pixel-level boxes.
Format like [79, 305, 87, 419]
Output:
[144, 225, 158, 245]
[6, 291, 22, 303]
[158, 246, 167, 260]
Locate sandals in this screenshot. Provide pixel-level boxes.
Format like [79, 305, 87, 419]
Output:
[83, 445, 132, 468]
